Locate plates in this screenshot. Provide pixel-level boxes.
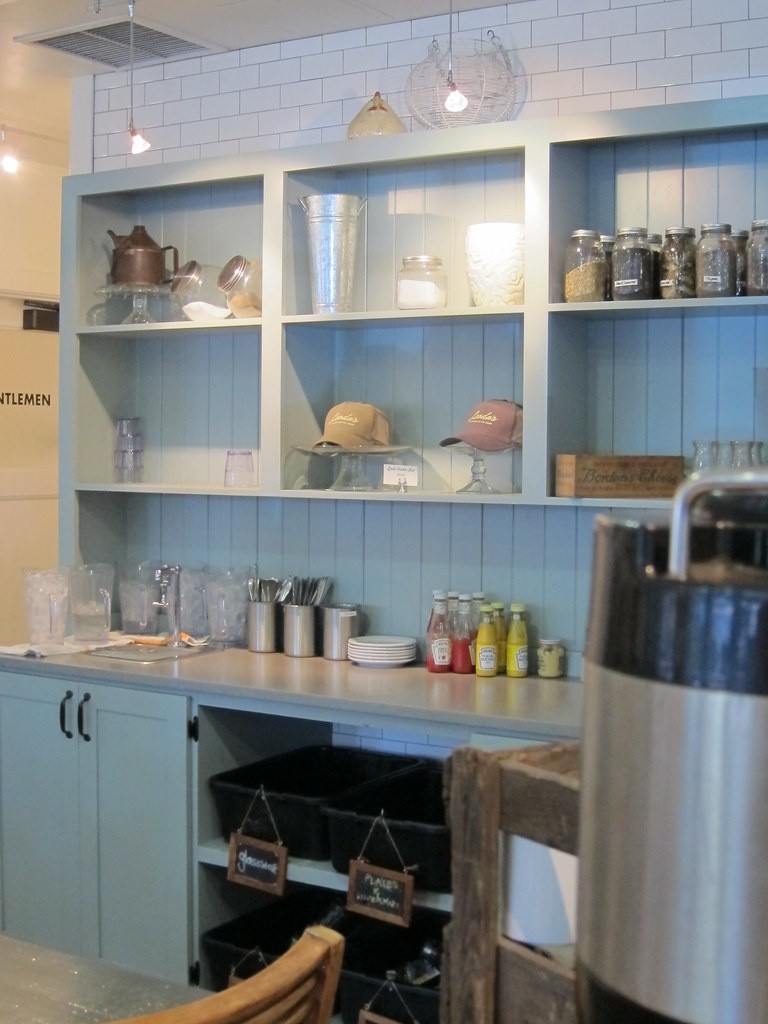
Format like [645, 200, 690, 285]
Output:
[346, 635, 417, 667]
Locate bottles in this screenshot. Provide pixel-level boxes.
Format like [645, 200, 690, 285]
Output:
[426, 589, 529, 677]
[536, 638, 565, 677]
[216, 255, 262, 318]
[170, 261, 233, 321]
[562, 217, 768, 303]
[681, 441, 767, 518]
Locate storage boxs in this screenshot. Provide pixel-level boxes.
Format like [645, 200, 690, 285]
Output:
[319, 761, 450, 892]
[338, 906, 452, 1024]
[207, 742, 427, 863]
[197, 891, 381, 1014]
[554, 453, 683, 499]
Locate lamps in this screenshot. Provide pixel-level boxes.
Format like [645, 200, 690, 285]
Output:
[443, 1, 469, 111]
[128, 0, 151, 154]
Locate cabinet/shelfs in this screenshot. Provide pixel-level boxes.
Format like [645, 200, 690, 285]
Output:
[0, 96, 767, 991]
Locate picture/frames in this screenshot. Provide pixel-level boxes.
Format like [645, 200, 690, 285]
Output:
[347, 860, 413, 928]
[226, 833, 287, 896]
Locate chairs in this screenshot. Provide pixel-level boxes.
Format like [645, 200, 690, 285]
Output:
[104, 921, 344, 1024]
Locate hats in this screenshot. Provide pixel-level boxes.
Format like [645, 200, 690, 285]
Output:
[311, 401, 389, 448]
[439, 398, 522, 451]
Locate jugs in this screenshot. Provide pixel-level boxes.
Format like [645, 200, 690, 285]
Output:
[23, 556, 256, 649]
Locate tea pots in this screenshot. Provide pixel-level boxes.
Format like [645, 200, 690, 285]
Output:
[105, 225, 180, 289]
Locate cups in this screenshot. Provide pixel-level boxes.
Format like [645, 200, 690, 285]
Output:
[463, 222, 525, 310]
[224, 450, 256, 489]
[245, 599, 366, 660]
[113, 417, 144, 484]
[395, 254, 446, 310]
